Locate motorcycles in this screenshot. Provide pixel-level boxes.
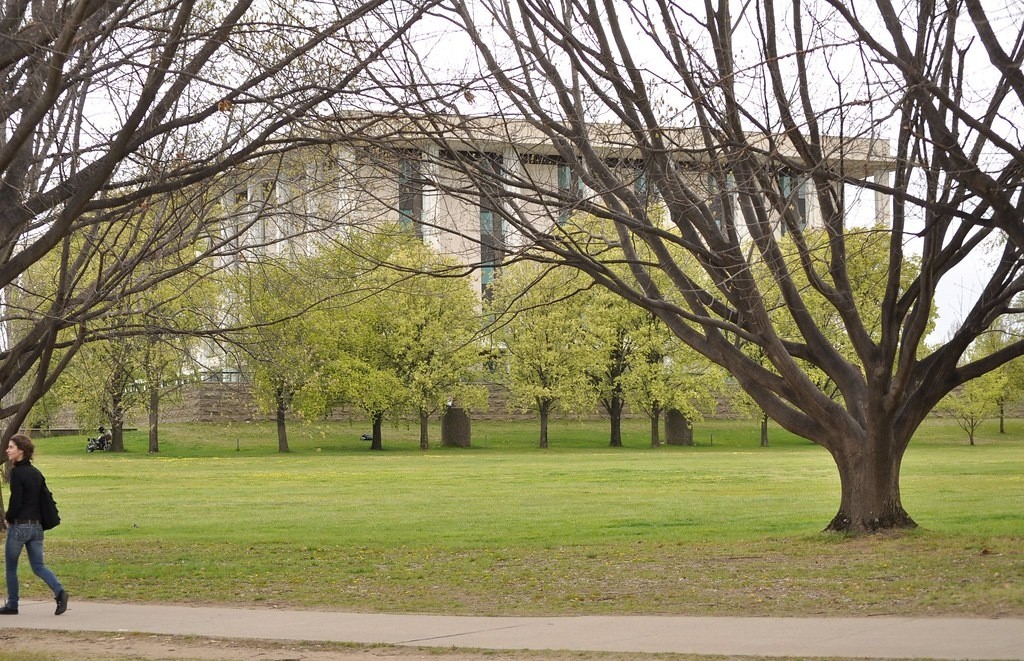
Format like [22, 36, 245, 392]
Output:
[86, 427, 113, 453]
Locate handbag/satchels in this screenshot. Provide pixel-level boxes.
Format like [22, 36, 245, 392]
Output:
[39, 483, 60, 531]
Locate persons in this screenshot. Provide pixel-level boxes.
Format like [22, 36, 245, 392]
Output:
[0, 434, 69, 615]
[98, 425, 113, 449]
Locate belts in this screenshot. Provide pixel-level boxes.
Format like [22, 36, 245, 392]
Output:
[10, 517, 40, 524]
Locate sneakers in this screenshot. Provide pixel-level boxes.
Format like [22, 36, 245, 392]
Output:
[0, 601, 18, 614]
[55, 590, 68, 615]
